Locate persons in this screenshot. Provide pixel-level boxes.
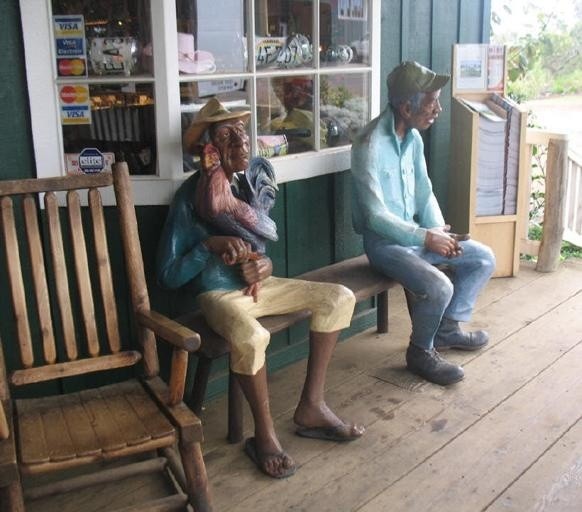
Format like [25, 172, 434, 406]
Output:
[349, 61, 495, 387]
[153, 94, 367, 479]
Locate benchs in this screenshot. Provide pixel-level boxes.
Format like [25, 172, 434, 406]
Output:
[175, 251, 450, 441]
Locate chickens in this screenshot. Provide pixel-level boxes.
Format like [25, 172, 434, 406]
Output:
[194, 143, 280, 302]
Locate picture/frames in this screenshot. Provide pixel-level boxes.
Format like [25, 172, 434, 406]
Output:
[336, 0, 368, 22]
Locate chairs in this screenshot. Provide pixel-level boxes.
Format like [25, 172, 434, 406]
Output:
[0, 161, 217, 511]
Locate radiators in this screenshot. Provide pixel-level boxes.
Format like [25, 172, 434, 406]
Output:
[88, 104, 144, 144]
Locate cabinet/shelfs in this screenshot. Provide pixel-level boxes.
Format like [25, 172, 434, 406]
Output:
[20, 2, 382, 176]
